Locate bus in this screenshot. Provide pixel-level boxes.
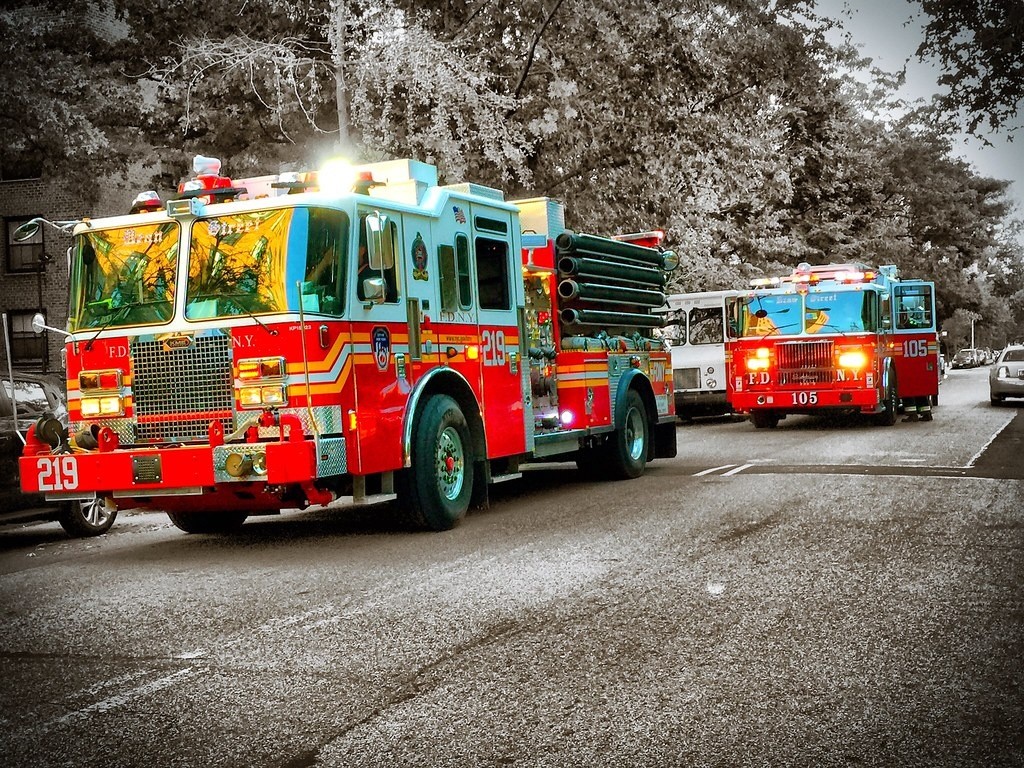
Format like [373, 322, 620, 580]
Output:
[652, 290, 747, 422]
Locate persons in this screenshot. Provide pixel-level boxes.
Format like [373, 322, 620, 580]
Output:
[902, 397, 933, 422]
[307, 235, 390, 302]
[939, 337, 948, 385]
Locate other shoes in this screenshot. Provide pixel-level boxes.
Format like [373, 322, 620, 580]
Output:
[902, 412, 934, 422]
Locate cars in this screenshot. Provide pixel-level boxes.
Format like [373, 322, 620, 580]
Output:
[990, 345, 1024, 406]
[951, 348, 1003, 368]
[0, 368, 118, 538]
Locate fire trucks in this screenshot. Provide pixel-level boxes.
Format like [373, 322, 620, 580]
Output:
[720, 261, 940, 428]
[18, 157, 680, 535]
